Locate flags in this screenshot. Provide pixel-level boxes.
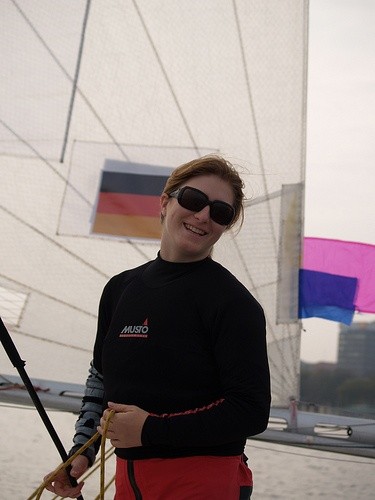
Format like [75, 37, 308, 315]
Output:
[298, 269, 359, 328]
[301, 235, 375, 314]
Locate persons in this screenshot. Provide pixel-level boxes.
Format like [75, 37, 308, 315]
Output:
[42, 152, 273, 500]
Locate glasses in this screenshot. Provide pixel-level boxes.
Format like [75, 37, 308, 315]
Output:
[169, 185, 235, 227]
